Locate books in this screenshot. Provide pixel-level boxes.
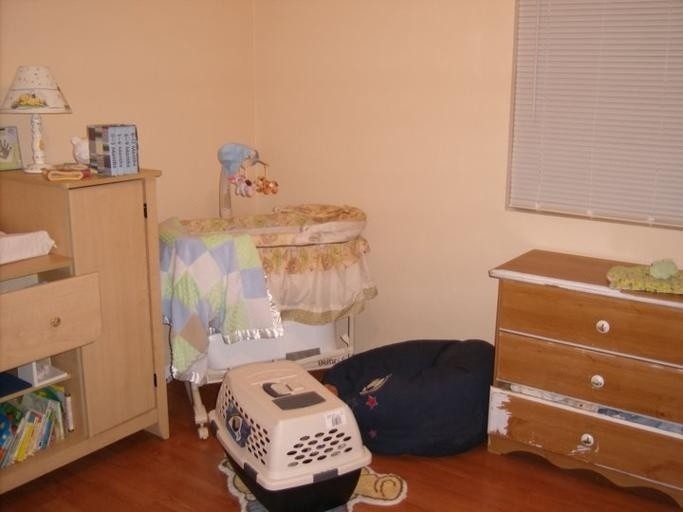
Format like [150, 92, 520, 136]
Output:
[0, 383, 73, 468]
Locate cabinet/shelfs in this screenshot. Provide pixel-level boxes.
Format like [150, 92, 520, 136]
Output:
[0, 165, 169, 500]
[489, 248, 683, 508]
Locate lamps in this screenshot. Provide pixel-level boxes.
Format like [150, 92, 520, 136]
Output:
[0, 64, 72, 174]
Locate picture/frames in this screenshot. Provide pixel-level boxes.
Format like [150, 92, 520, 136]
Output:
[0, 125, 24, 171]
